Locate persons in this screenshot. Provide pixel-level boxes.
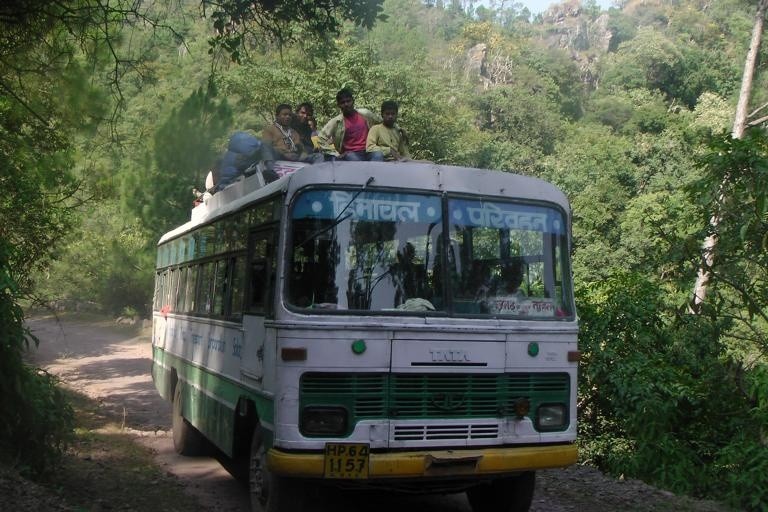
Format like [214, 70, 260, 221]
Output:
[262, 102, 318, 184]
[496, 260, 526, 297]
[318, 89, 409, 162]
[366, 101, 410, 163]
[293, 239, 369, 308]
[390, 242, 433, 308]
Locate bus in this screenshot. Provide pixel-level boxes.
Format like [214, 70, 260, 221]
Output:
[144, 162, 582, 512]
